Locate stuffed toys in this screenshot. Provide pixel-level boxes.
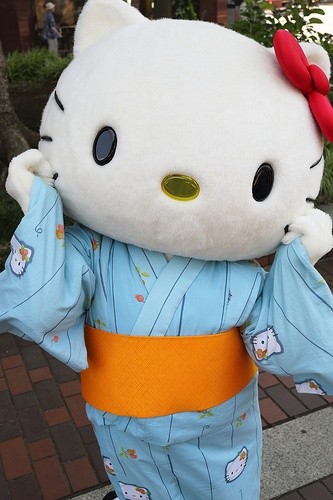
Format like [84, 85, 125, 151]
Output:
[0, 0, 333, 499]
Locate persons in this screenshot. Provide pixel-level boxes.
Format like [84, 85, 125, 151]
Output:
[43, 1, 61, 58]
